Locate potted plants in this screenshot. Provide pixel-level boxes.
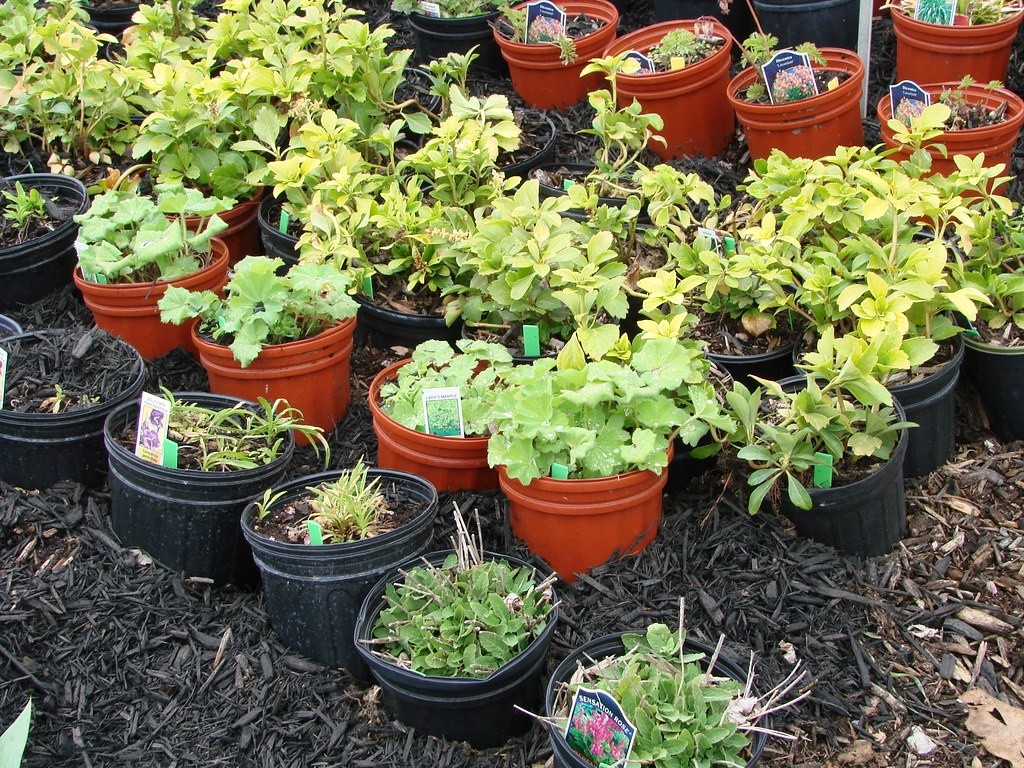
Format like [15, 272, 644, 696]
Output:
[0, 0, 1024, 768]
